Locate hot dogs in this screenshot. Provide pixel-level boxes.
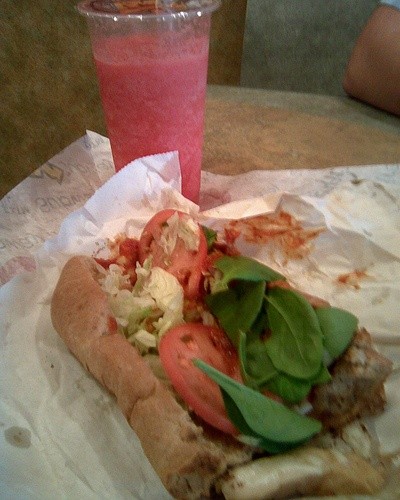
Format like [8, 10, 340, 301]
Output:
[46, 209, 400, 500]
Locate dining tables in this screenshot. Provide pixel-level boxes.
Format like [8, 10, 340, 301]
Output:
[201, 84, 400, 178]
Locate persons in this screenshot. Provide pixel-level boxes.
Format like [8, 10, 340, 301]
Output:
[341, 0, 400, 118]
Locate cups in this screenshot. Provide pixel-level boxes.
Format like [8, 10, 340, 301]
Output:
[75, 0, 222, 204]
